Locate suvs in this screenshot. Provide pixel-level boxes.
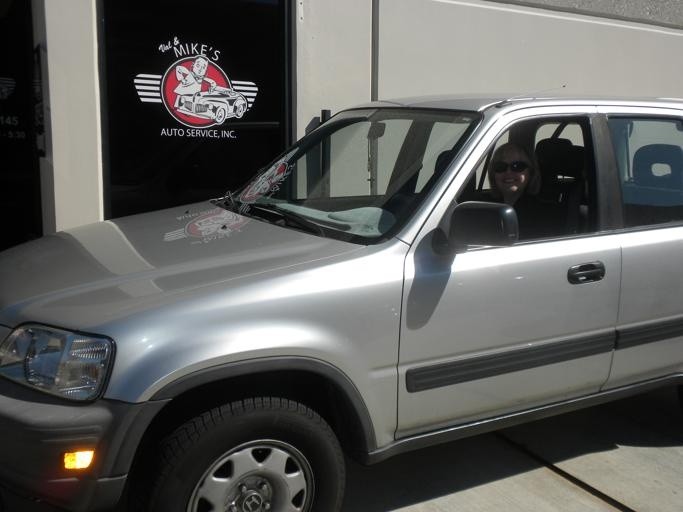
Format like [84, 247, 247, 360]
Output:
[0, 98, 682, 511]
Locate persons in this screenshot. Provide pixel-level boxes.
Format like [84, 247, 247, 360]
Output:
[487, 142, 568, 241]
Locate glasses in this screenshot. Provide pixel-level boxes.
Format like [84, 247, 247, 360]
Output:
[493, 161, 528, 174]
